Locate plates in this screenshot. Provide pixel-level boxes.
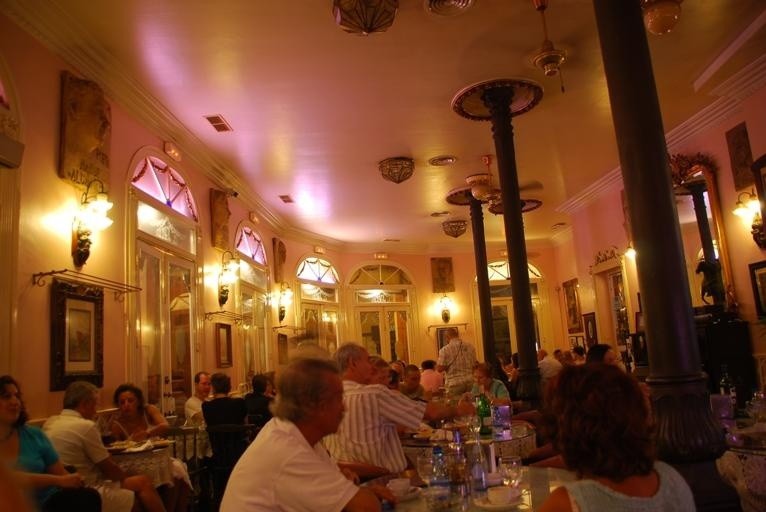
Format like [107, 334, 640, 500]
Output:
[413, 428, 435, 439]
[382, 486, 421, 503]
[474, 495, 524, 511]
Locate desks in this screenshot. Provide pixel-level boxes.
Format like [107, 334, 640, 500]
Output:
[103, 392, 766, 512]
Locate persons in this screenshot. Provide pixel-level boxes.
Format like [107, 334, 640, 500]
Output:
[508, 343, 695, 511]
[437, 328, 479, 393]
[184, 372, 273, 464]
[220, 342, 474, 511]
[1, 375, 180, 512]
[462, 363, 509, 403]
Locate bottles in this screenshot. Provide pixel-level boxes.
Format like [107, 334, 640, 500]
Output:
[428, 429, 488, 512]
[477, 384, 492, 435]
[720, 363, 736, 420]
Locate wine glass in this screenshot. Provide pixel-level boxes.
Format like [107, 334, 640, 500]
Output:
[709, 395, 736, 443]
[468, 416, 481, 443]
[417, 457, 439, 497]
[745, 391, 766, 426]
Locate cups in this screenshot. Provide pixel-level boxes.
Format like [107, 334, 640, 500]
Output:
[386, 478, 411, 496]
[498, 456, 521, 487]
[487, 486, 512, 504]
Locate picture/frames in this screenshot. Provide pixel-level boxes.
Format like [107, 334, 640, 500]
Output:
[278, 333, 289, 365]
[216, 323, 233, 368]
[562, 278, 584, 334]
[437, 327, 458, 357]
[50, 289, 104, 392]
[750, 260, 766, 320]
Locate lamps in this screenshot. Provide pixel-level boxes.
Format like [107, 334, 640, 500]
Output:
[72, 179, 114, 267]
[433, 287, 457, 321]
[640, 0, 684, 35]
[466, 175, 495, 200]
[218, 250, 240, 305]
[279, 282, 293, 321]
[732, 188, 766, 248]
